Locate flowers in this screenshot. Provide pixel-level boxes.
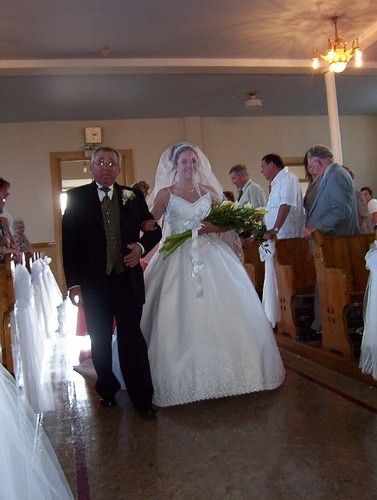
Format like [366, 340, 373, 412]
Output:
[121, 189, 136, 205]
[159, 201, 272, 260]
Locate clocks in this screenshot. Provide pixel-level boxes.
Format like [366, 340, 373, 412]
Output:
[85, 127, 102, 144]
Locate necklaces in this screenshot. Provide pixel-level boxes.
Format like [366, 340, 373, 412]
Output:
[173, 183, 199, 193]
[178, 184, 200, 192]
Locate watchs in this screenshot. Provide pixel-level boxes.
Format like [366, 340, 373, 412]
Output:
[273, 227, 280, 233]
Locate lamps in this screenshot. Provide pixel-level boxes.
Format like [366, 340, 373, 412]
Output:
[245, 91, 262, 111]
[311, 16, 363, 74]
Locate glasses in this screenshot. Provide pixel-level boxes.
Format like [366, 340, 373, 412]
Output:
[0, 198, 6, 203]
[92, 160, 119, 167]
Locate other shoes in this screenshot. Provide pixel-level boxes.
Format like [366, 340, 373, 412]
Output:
[81, 351, 91, 359]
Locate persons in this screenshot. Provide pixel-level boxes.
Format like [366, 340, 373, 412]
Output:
[297, 144, 359, 340]
[11, 220, 34, 273]
[131, 180, 150, 198]
[261, 154, 306, 238]
[360, 186, 377, 233]
[229, 164, 266, 207]
[0, 177, 22, 266]
[303, 152, 320, 213]
[222, 191, 236, 203]
[124, 143, 286, 408]
[61, 147, 162, 417]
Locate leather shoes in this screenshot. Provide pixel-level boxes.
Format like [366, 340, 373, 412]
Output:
[100, 397, 116, 407]
[139, 405, 156, 418]
[299, 331, 322, 341]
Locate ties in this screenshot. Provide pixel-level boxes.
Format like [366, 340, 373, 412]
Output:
[238, 190, 243, 202]
[102, 187, 111, 210]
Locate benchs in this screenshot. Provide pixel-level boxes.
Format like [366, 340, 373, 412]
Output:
[242, 234, 377, 359]
